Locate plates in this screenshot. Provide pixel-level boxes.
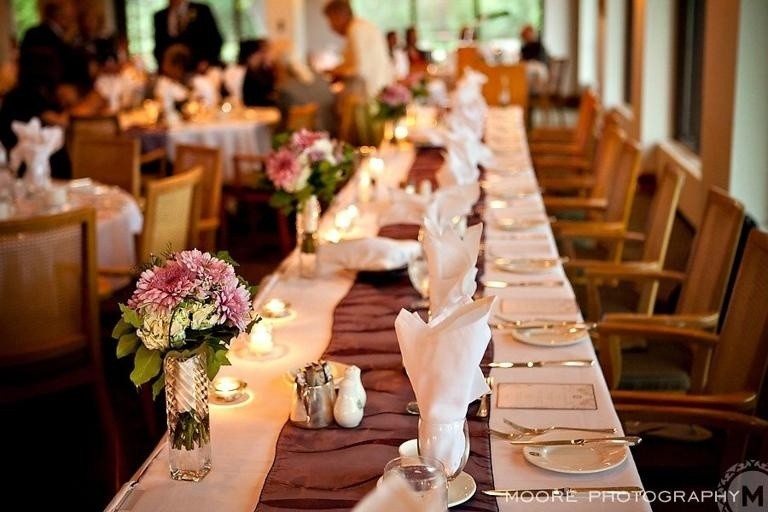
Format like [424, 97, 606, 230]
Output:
[323, 359, 353, 389]
[485, 103, 590, 347]
[522, 426, 630, 476]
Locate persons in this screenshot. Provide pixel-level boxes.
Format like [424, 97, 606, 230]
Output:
[387, 29, 410, 69]
[454, 25, 487, 63]
[404, 26, 427, 63]
[519, 23, 554, 65]
[1, 0, 394, 186]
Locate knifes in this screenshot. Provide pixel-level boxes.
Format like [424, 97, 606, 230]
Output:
[482, 357, 596, 371]
[510, 434, 645, 450]
[480, 485, 645, 499]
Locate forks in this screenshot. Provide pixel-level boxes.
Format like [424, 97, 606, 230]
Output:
[477, 375, 492, 417]
[491, 417, 617, 439]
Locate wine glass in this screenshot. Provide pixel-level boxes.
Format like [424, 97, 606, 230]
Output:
[406, 252, 432, 310]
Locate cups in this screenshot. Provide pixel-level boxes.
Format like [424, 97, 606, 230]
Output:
[288, 373, 338, 432]
[210, 297, 292, 400]
[380, 419, 477, 511]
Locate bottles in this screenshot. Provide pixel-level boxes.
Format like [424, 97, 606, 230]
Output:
[334, 366, 369, 427]
